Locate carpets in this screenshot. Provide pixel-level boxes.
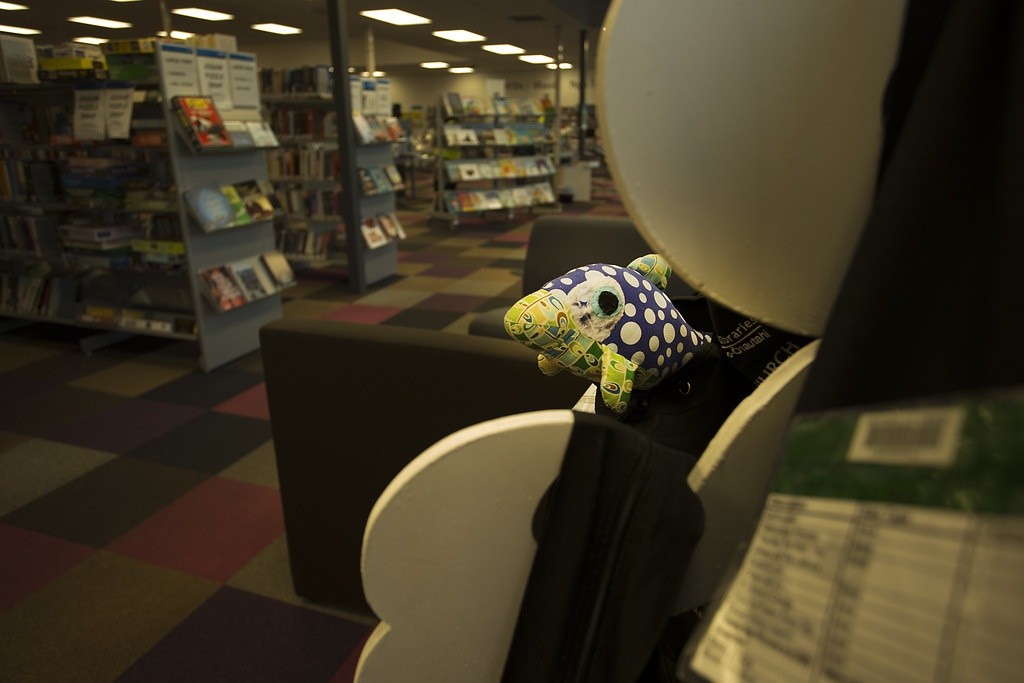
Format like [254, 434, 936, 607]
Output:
[116, 582, 378, 682]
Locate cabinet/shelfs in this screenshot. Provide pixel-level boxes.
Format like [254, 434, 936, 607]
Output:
[0, 36, 602, 372]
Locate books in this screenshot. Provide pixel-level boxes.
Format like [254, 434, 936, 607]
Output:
[440, 92, 555, 213]
[0, 33, 407, 335]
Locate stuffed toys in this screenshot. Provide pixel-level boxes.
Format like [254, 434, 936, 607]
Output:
[504, 253, 713, 414]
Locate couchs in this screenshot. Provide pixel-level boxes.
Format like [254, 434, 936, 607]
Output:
[260, 212, 703, 600]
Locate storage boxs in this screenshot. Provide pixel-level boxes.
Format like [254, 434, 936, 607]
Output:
[58, 226, 138, 243]
[100, 39, 185, 56]
[106, 53, 160, 80]
